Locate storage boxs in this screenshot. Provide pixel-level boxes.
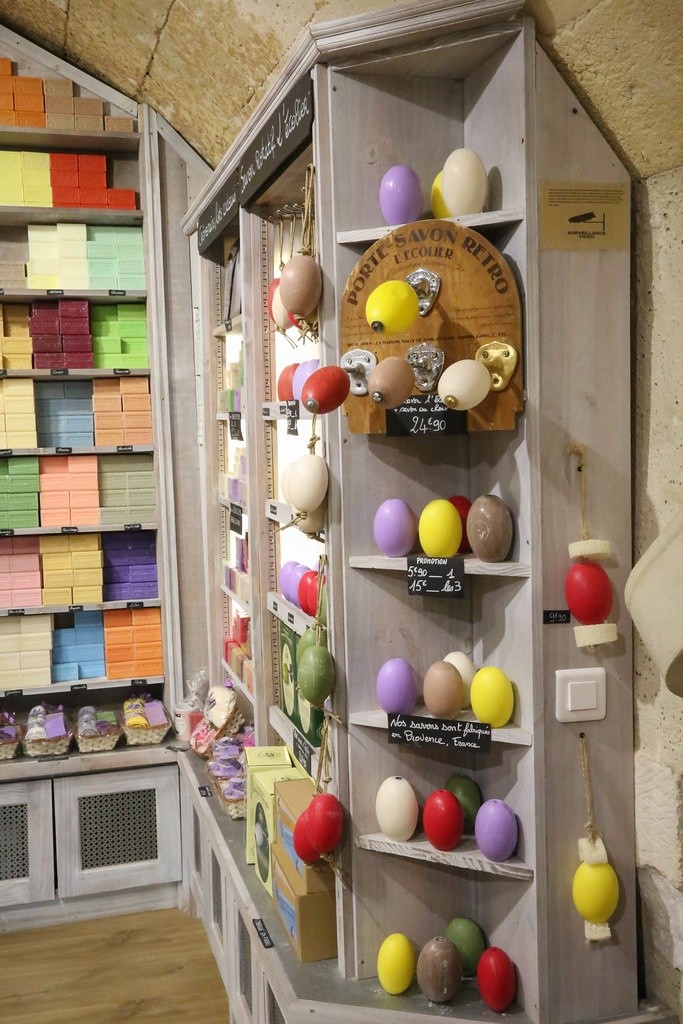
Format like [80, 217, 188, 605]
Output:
[0, 223, 147, 290]
[0, 454, 157, 528]
[0, 299, 148, 370]
[271, 843, 338, 963]
[295, 634, 324, 748]
[0, 56, 134, 132]
[0, 375, 154, 449]
[278, 621, 298, 728]
[273, 779, 336, 893]
[0, 150, 137, 210]
[242, 745, 293, 866]
[174, 0, 646, 1024]
[0, 530, 164, 689]
[252, 767, 305, 899]
[216, 340, 255, 694]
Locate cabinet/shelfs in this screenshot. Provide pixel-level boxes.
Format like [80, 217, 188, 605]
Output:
[0, 101, 186, 935]
